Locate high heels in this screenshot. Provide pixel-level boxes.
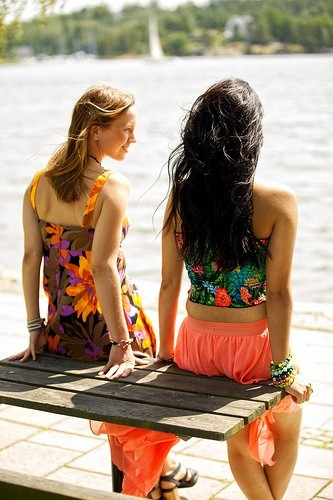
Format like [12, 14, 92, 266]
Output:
[159, 462, 199, 500]
[148, 486, 161, 500]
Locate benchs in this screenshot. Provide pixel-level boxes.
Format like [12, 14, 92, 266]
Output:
[0, 350, 289, 493]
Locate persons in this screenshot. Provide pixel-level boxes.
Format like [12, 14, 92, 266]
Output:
[156, 78, 313, 500]
[9, 84, 197, 500]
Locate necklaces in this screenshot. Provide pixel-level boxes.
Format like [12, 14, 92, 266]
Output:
[87, 154, 103, 169]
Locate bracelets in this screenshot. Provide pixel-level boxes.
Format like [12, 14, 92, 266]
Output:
[110, 337, 133, 351]
[157, 354, 174, 363]
[270, 352, 296, 388]
[27, 318, 46, 332]
